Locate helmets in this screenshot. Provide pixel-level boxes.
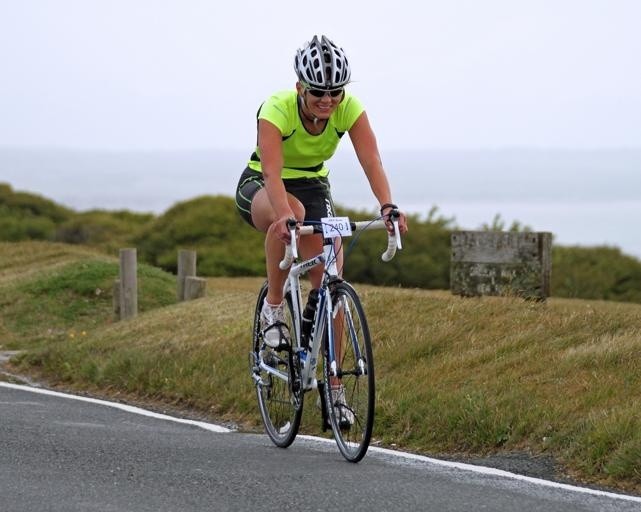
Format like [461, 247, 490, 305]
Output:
[294, 34, 351, 91]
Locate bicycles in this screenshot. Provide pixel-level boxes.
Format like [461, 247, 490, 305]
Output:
[244, 210, 404, 462]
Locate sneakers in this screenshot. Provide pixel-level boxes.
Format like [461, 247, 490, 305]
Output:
[316, 384, 354, 429]
[260, 297, 291, 348]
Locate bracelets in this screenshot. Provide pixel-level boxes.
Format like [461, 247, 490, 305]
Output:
[380, 204, 398, 215]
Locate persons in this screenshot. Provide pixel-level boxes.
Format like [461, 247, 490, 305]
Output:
[235, 34, 408, 425]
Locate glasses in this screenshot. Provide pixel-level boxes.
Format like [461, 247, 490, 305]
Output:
[309, 90, 342, 97]
